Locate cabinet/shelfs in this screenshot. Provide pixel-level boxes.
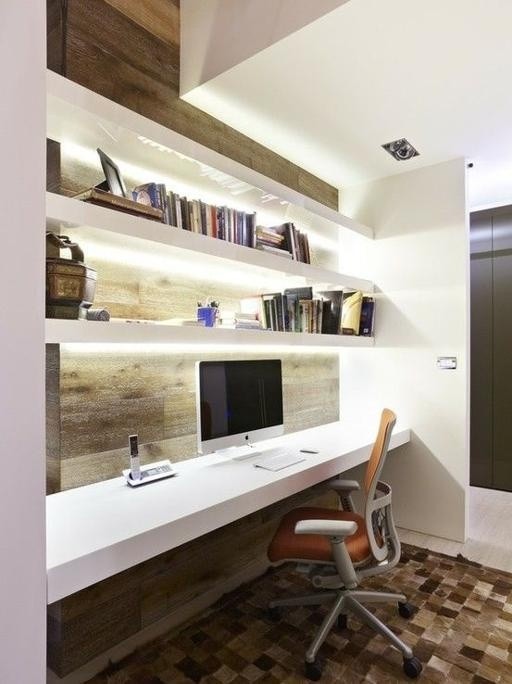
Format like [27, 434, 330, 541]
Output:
[46, 59, 411, 358]
[469, 203, 512, 493]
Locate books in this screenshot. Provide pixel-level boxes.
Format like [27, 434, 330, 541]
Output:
[130, 175, 311, 267]
[236, 286, 375, 339]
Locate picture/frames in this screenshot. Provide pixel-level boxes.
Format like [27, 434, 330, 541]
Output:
[97, 149, 126, 200]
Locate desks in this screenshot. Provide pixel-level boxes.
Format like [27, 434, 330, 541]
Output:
[44, 414, 414, 608]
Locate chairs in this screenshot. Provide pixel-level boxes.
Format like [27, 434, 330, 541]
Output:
[268, 409, 422, 680]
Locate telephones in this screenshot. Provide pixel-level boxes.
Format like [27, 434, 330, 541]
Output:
[122, 434, 176, 486]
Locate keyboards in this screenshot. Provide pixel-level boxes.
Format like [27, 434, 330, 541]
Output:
[254, 450, 305, 473]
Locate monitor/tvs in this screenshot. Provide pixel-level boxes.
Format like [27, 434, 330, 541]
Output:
[195, 359, 285, 460]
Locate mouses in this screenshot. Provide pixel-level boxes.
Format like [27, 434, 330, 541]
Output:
[299, 447, 319, 453]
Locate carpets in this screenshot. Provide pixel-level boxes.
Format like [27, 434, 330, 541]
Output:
[86, 543, 512, 684]
